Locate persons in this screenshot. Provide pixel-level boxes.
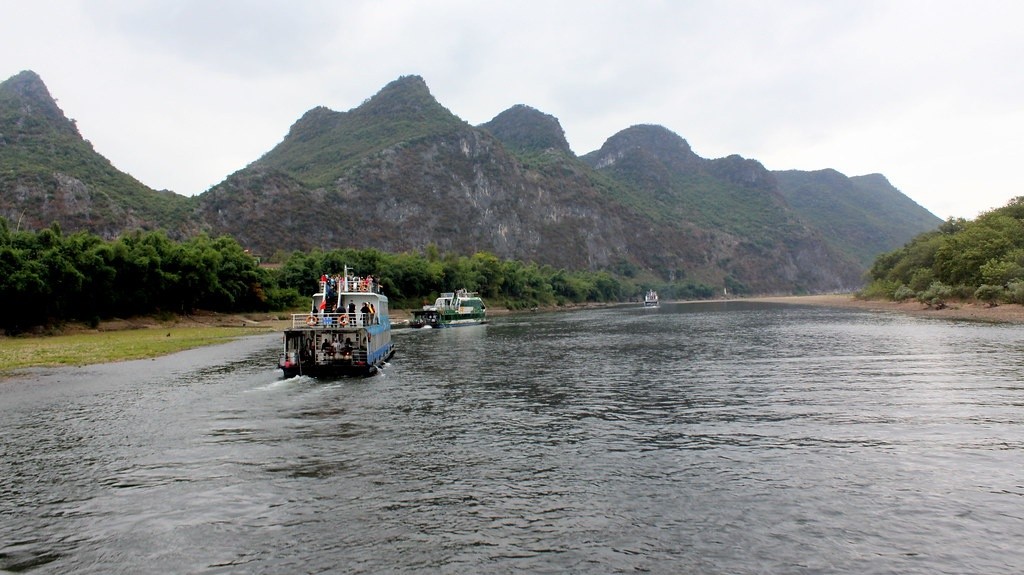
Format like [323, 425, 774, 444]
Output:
[320, 272, 380, 292]
[310, 300, 376, 328]
[300, 330, 367, 364]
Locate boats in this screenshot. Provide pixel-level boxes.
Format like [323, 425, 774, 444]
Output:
[645, 289, 658, 306]
[409, 289, 487, 330]
[278, 267, 395, 379]
